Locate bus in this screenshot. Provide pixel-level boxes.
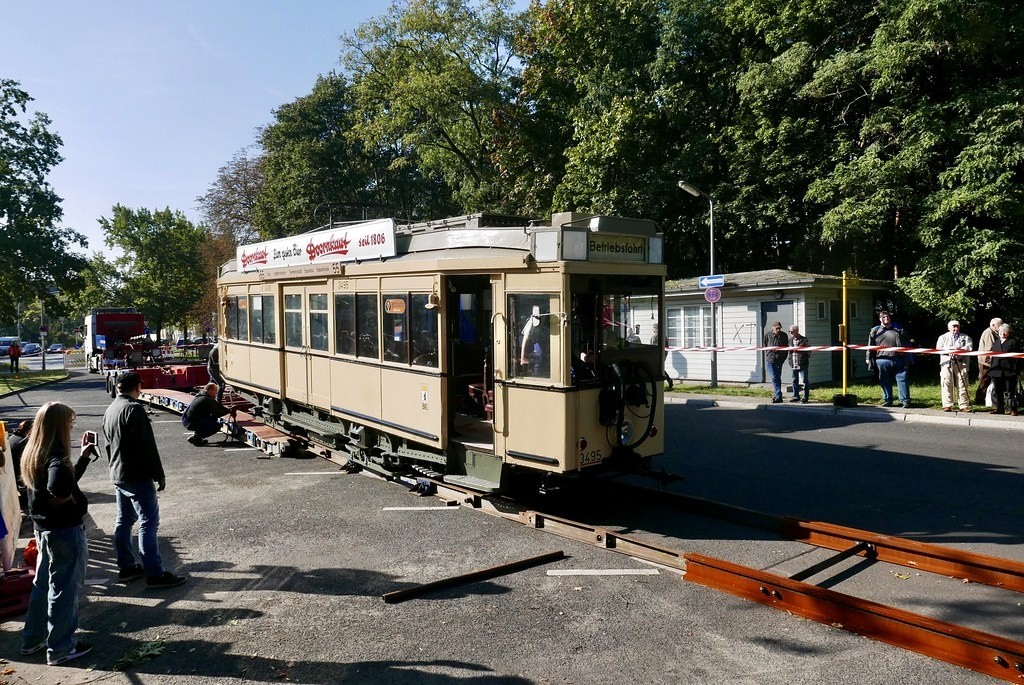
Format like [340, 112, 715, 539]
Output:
[214, 209, 666, 498]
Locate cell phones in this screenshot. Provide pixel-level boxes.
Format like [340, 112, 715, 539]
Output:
[85, 431, 98, 446]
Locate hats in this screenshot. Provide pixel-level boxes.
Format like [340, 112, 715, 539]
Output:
[947, 320, 960, 329]
[115, 373, 146, 389]
[772, 321, 782, 329]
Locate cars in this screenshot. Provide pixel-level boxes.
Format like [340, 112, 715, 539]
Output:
[21, 342, 28, 353]
[157, 339, 173, 345]
[22, 343, 40, 354]
[193, 337, 213, 343]
[177, 339, 193, 348]
[46, 343, 64, 354]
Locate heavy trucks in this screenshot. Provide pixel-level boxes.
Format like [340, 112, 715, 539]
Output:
[82, 308, 299, 456]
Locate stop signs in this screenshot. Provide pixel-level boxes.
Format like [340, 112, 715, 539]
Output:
[73, 327, 79, 333]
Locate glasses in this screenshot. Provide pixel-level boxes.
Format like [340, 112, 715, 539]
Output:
[788, 330, 795, 333]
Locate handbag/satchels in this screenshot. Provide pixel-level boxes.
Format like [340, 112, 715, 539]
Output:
[868, 355, 876, 371]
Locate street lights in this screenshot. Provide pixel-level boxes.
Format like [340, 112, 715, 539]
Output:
[41, 290, 59, 370]
[677, 179, 718, 388]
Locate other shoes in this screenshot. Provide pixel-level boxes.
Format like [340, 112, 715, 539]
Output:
[145, 571, 186, 587]
[801, 398, 809, 403]
[790, 398, 800, 402]
[118, 564, 145, 581]
[944, 407, 951, 412]
[960, 407, 972, 412]
[1010, 410, 1018, 416]
[771, 396, 783, 403]
[882, 401, 893, 406]
[187, 434, 208, 447]
[989, 410, 997, 414]
[902, 401, 910, 408]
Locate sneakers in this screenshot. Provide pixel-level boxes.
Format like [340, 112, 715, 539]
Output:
[47, 640, 93, 665]
[21, 636, 48, 654]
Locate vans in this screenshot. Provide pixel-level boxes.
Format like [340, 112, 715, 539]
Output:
[0, 336, 21, 357]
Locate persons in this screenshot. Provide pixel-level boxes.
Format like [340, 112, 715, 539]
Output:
[787, 325, 812, 403]
[973, 318, 1022, 416]
[182, 383, 237, 447]
[201, 332, 218, 344]
[145, 333, 152, 344]
[764, 321, 789, 403]
[625, 327, 641, 344]
[101, 372, 187, 590]
[865, 311, 914, 408]
[0, 448, 9, 540]
[520, 298, 550, 378]
[936, 320, 972, 411]
[8, 341, 21, 373]
[20, 402, 93, 666]
[9, 421, 34, 535]
[233, 325, 439, 367]
[207, 343, 226, 404]
[650, 323, 673, 388]
